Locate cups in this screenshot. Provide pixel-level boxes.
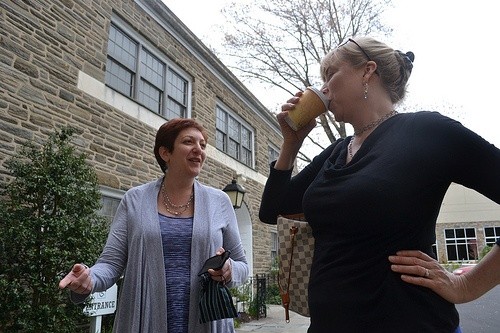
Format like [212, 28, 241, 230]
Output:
[284, 86, 329, 132]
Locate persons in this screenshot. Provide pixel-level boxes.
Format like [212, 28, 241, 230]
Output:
[259, 36, 500, 333]
[58, 118, 249, 333]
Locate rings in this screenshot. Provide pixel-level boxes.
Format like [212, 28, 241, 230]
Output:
[425, 268, 429, 278]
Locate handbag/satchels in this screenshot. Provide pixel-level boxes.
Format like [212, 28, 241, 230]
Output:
[277, 214, 310, 324]
[197, 274, 238, 325]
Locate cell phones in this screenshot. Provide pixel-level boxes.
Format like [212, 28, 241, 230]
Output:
[197, 250, 231, 277]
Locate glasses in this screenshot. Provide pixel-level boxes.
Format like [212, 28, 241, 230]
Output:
[331, 37, 381, 78]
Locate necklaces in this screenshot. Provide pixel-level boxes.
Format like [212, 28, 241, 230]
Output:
[349, 110, 398, 158]
[161, 183, 194, 216]
